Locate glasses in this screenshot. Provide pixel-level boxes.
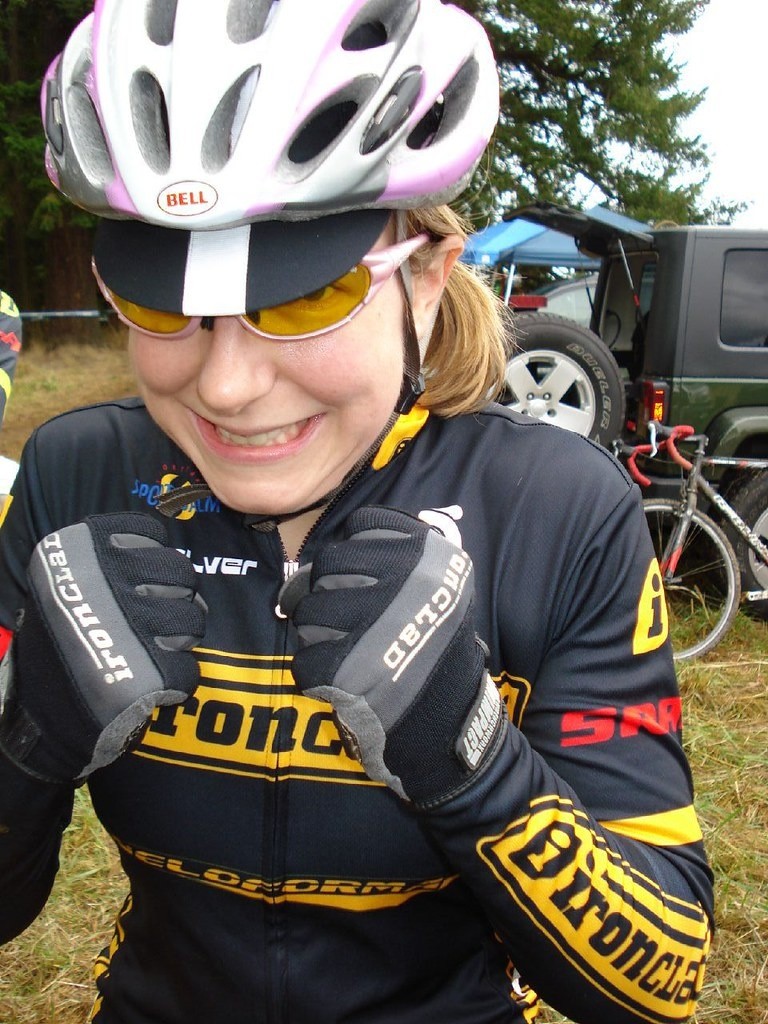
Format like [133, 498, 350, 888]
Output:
[90, 231, 428, 341]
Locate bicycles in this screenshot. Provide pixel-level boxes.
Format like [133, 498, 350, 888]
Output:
[596, 420, 768, 661]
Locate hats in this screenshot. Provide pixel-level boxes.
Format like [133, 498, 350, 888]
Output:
[92, 18, 443, 317]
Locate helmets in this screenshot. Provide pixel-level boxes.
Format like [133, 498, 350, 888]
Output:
[40, 0, 499, 230]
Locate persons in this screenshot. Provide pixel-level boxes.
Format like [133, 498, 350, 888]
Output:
[3, 0, 710, 1024]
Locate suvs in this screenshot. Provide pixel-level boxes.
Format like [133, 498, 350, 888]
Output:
[497, 198, 767, 623]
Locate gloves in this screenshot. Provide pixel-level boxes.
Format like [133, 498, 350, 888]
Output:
[276, 505, 508, 809]
[1, 510, 208, 785]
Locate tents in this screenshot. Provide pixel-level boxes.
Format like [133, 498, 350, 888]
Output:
[457, 205, 654, 311]
